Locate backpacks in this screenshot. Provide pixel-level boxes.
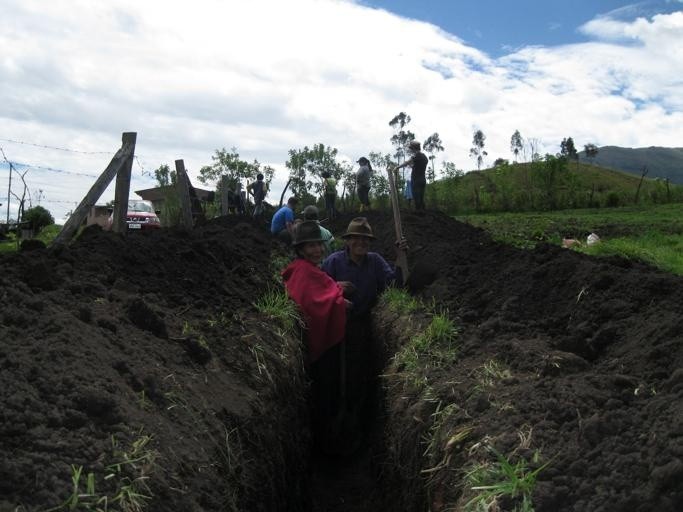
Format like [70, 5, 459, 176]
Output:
[326, 178, 336, 195]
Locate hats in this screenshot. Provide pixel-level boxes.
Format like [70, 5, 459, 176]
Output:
[409, 141, 421, 150]
[301, 205, 319, 220]
[289, 220, 328, 247]
[356, 157, 367, 163]
[342, 216, 377, 240]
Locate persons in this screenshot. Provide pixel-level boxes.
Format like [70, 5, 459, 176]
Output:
[356, 157, 373, 213]
[316, 172, 337, 221]
[269, 197, 411, 457]
[247, 174, 267, 220]
[392, 142, 428, 217]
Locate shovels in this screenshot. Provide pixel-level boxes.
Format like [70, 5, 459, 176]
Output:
[326, 306, 362, 455]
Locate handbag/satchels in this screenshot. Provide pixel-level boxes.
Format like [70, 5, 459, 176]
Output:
[359, 176, 369, 187]
[254, 189, 263, 205]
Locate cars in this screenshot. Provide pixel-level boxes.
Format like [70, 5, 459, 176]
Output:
[107, 200, 161, 232]
